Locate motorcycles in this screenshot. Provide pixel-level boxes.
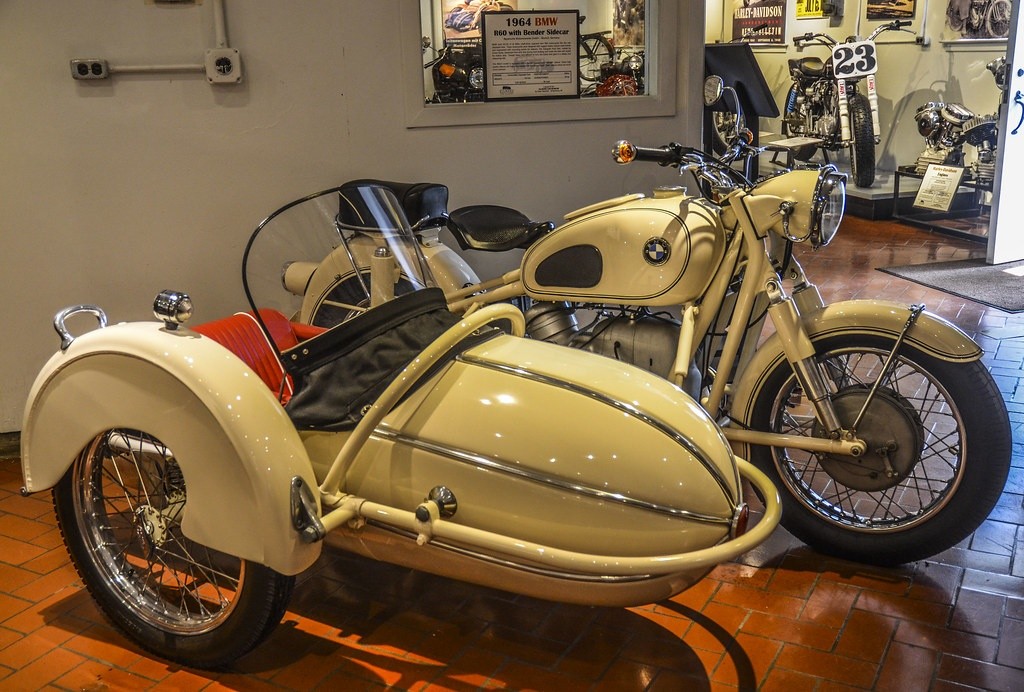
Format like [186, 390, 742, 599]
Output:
[947, 0, 1013, 38]
[20, 75, 1014, 672]
[782, 18, 917, 187]
[423, 28, 646, 103]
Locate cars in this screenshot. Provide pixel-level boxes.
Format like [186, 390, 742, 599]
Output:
[867, 0, 915, 18]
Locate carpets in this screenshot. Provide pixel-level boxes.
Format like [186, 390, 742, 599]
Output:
[872, 259, 1024, 314]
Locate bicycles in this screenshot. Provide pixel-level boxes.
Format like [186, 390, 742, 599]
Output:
[578, 14, 617, 81]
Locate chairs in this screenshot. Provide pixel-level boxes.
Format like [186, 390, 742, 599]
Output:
[194, 308, 303, 407]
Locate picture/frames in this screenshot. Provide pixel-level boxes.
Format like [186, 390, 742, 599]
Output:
[867, 0, 916, 19]
[796, 0, 829, 20]
